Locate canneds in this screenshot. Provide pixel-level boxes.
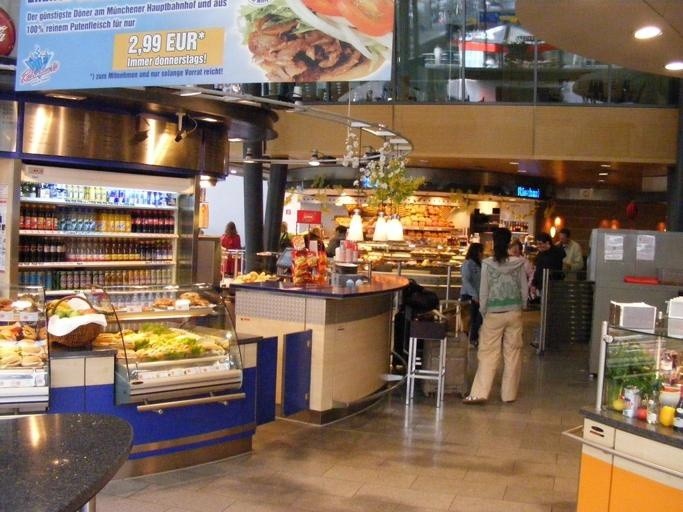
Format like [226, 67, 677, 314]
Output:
[17, 268, 172, 290]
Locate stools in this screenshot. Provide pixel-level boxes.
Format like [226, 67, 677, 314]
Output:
[404, 318, 449, 408]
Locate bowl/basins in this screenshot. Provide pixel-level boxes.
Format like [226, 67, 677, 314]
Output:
[659, 386, 681, 405]
[335, 262, 358, 273]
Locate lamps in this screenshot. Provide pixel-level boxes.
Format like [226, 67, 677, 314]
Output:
[344, 127, 365, 242]
[374, 135, 406, 243]
[131, 113, 150, 133]
[308, 148, 320, 168]
[173, 109, 200, 142]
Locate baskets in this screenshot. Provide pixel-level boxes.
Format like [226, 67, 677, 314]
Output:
[51, 295, 101, 348]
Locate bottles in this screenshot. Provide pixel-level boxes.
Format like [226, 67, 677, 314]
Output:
[17, 181, 175, 307]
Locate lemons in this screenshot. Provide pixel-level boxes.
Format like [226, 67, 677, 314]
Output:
[659, 406, 675, 427]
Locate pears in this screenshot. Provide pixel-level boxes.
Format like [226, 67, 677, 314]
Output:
[613, 399, 624, 411]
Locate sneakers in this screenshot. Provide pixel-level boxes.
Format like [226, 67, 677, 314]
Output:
[461, 395, 488, 405]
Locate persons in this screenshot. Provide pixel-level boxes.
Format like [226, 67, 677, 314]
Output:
[461, 229, 529, 404]
[458, 229, 584, 347]
[218, 222, 241, 278]
[279, 222, 349, 259]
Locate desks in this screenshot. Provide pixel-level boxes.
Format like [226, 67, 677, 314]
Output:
[0, 411, 133, 512]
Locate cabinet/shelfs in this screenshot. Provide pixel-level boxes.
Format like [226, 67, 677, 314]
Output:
[0, 284, 52, 413]
[88, 284, 247, 415]
[15, 178, 180, 308]
[561, 320, 683, 512]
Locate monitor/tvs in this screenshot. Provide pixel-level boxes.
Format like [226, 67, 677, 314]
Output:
[515, 184, 541, 198]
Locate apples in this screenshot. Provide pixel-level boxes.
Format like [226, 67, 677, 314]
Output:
[636, 406, 647, 420]
